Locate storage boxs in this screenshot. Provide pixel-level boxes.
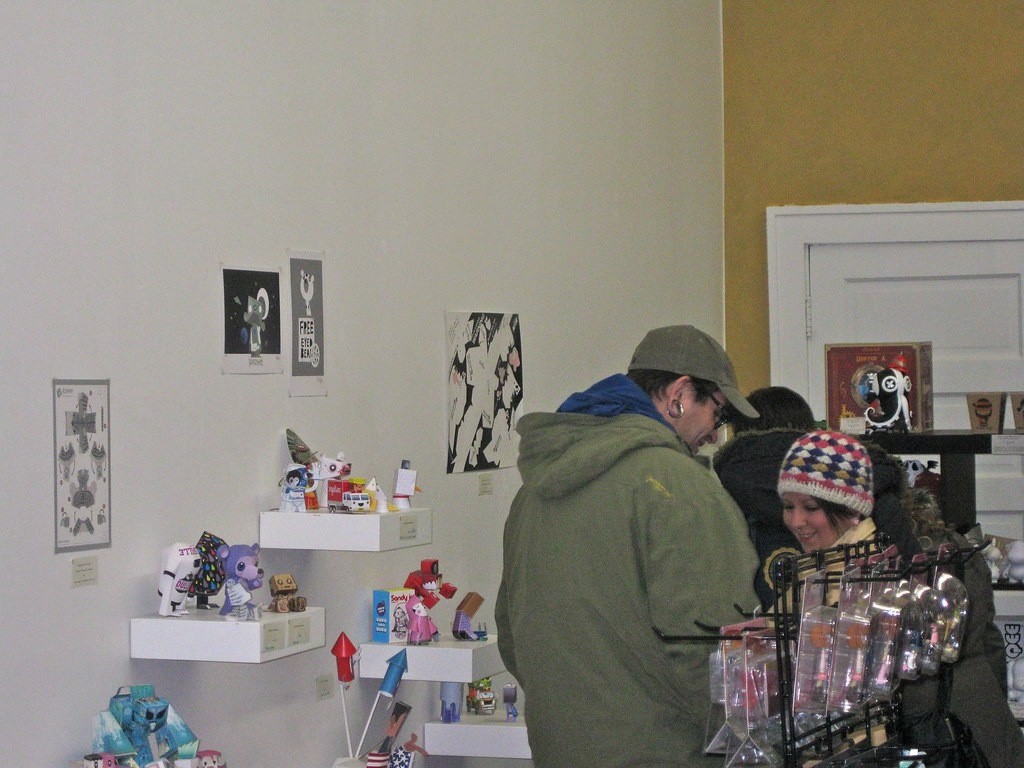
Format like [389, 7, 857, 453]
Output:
[823, 341, 934, 436]
[964, 390, 1007, 434]
[1010, 391, 1024, 433]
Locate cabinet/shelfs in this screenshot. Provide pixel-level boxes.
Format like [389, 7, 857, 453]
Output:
[130, 507, 533, 760]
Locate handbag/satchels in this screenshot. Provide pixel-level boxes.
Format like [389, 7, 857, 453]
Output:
[864, 708, 991, 768]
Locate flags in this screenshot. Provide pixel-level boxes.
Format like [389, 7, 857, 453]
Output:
[366, 745, 414, 768]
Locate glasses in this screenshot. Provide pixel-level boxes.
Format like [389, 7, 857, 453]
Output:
[701, 385, 734, 429]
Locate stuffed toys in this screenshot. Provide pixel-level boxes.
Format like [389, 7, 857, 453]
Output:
[215, 542, 265, 614]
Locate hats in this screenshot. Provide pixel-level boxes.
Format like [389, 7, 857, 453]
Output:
[777, 430, 874, 517]
[628, 325, 761, 418]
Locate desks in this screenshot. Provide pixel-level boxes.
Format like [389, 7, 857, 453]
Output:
[821, 428, 993, 534]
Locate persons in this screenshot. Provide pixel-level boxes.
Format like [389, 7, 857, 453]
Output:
[494, 324, 1024, 768]
